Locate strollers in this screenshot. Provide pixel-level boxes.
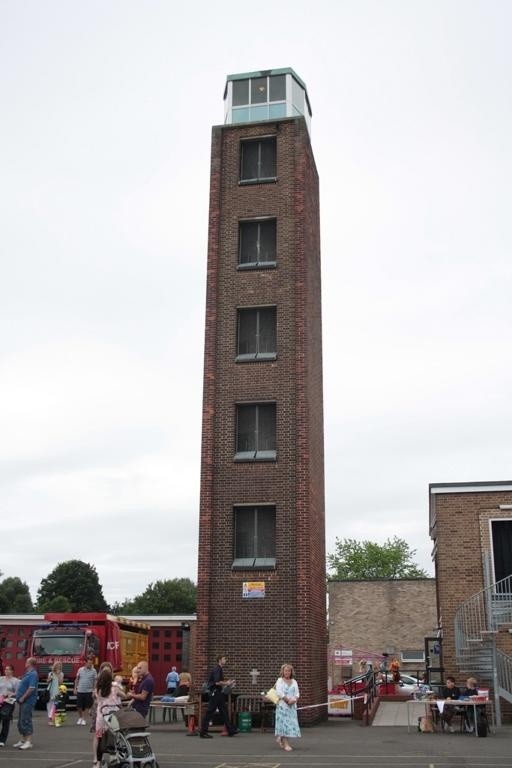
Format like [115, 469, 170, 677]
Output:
[99, 703, 162, 767]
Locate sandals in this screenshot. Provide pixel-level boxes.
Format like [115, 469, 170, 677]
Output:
[276, 737, 293, 752]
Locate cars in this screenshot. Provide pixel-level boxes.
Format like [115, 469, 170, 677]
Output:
[380, 671, 430, 699]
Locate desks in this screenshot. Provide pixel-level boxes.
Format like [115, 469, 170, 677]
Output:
[146, 684, 279, 737]
[403, 695, 497, 738]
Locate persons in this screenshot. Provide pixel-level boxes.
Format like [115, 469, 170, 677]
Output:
[0, 665, 20, 747]
[439, 677, 460, 733]
[200, 655, 240, 739]
[73, 655, 154, 768]
[46, 661, 64, 726]
[52, 684, 69, 728]
[463, 677, 478, 733]
[166, 666, 179, 694]
[179, 667, 191, 696]
[359, 656, 401, 687]
[274, 664, 302, 752]
[12, 657, 38, 750]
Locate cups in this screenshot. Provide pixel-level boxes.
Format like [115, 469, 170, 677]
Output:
[416, 691, 422, 701]
[426, 691, 434, 702]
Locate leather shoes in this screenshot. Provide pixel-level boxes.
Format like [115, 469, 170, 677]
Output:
[228, 729, 241, 738]
[199, 731, 214, 740]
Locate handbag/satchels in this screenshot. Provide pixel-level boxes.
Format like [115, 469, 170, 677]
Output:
[38, 689, 50, 703]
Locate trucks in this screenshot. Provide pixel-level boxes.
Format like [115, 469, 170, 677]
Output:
[24, 611, 151, 710]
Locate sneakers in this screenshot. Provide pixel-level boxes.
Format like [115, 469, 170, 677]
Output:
[12, 740, 24, 748]
[47, 719, 65, 728]
[0, 742, 5, 747]
[19, 740, 33, 750]
[76, 717, 87, 726]
[444, 722, 474, 734]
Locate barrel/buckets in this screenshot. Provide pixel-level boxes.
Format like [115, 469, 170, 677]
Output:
[238, 711, 253, 732]
